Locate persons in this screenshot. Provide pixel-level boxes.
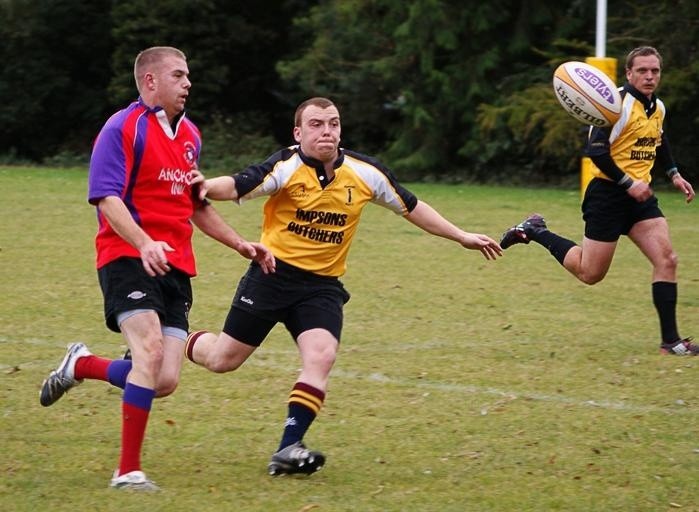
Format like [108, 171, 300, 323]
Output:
[500, 44, 699, 357]
[124, 97, 504, 477]
[36, 45, 280, 493]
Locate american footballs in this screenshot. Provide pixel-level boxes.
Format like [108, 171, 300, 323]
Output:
[552, 61, 622, 128]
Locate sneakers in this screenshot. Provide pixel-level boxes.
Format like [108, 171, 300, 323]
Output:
[40, 342, 94, 407]
[660, 335, 699, 356]
[500, 213, 547, 249]
[269, 441, 325, 476]
[109, 468, 161, 492]
